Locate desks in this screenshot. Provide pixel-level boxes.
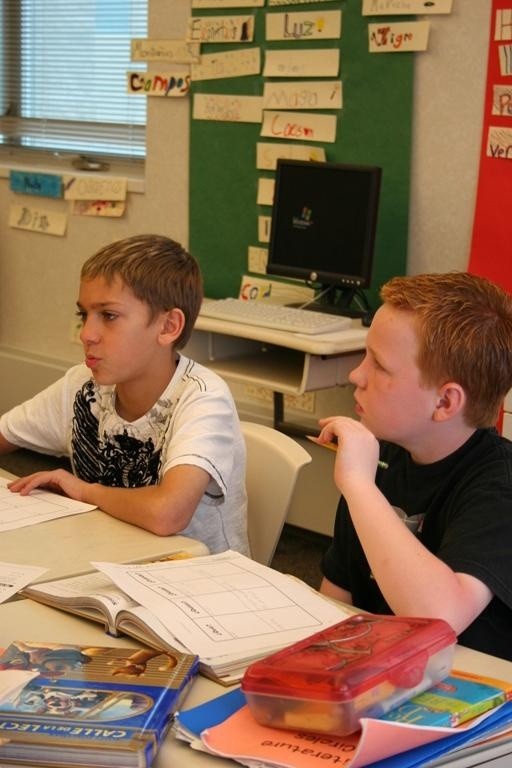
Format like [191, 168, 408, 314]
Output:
[0, 554, 512, 765]
[175, 296, 373, 428]
[0, 454, 211, 592]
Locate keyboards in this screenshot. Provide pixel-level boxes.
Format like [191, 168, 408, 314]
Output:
[199, 296, 352, 335]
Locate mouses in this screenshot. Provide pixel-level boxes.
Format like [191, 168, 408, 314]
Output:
[362, 313, 375, 328]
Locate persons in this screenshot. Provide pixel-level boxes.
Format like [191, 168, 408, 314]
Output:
[319, 273, 512, 663]
[2, 233, 254, 558]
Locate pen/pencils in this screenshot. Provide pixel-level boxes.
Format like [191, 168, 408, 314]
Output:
[305, 434, 390, 469]
[258, 660, 441, 731]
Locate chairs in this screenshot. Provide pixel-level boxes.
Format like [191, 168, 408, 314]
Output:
[238, 421, 313, 567]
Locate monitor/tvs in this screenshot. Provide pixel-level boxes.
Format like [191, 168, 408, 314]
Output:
[265, 158, 382, 322]
